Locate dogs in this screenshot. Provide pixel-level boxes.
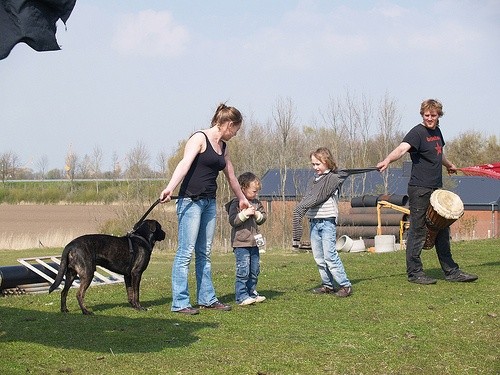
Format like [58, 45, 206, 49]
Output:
[49, 219, 166, 316]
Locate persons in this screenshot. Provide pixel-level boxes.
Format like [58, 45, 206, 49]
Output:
[376, 98, 479, 285]
[292, 147, 351, 297]
[159, 103, 253, 315]
[224, 172, 267, 306]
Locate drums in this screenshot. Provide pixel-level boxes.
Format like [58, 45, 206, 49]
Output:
[401, 189, 465, 251]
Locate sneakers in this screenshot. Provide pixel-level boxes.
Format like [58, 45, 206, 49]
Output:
[311, 285, 334, 294]
[409, 275, 437, 285]
[177, 307, 199, 314]
[445, 270, 478, 283]
[333, 286, 353, 297]
[253, 296, 266, 303]
[199, 302, 231, 310]
[239, 297, 255, 306]
